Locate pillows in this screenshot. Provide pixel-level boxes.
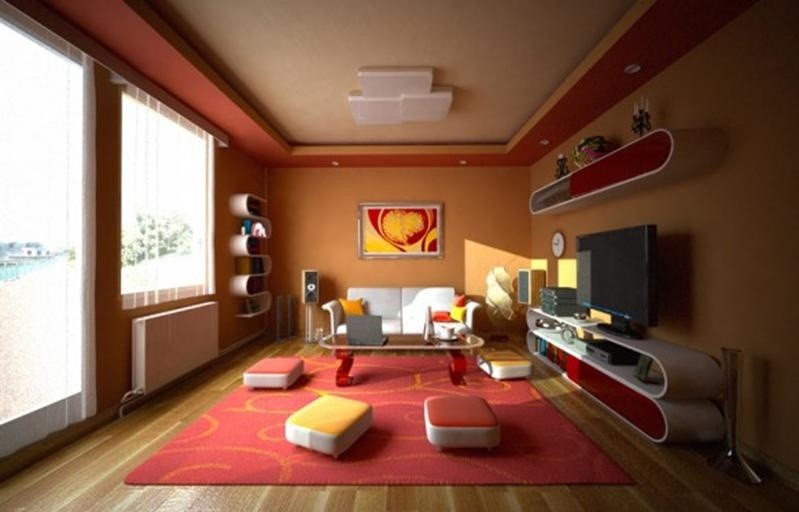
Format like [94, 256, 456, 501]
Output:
[338, 296, 367, 321]
[449, 303, 468, 324]
[453, 293, 465, 308]
[430, 309, 452, 324]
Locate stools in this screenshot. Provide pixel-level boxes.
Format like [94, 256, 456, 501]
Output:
[422, 396, 502, 454]
[242, 358, 306, 391]
[478, 349, 533, 383]
[286, 390, 373, 458]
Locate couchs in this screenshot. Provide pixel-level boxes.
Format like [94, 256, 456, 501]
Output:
[320, 286, 481, 354]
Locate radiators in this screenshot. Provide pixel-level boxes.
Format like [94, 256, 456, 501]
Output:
[130, 298, 222, 398]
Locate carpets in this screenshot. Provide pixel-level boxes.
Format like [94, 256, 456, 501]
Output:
[124, 354, 638, 485]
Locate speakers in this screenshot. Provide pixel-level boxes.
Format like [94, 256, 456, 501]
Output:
[302, 269, 319, 304]
[518, 269, 545, 305]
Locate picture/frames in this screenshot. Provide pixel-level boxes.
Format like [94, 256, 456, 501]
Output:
[357, 200, 445, 261]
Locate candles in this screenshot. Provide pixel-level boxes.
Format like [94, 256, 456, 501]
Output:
[558, 153, 565, 159]
[632, 91, 650, 117]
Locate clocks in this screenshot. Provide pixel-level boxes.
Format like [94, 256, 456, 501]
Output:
[551, 228, 567, 258]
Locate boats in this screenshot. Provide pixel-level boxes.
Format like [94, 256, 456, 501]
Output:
[0, 243, 73, 266]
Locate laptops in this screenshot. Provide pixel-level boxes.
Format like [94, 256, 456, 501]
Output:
[347, 316, 388, 346]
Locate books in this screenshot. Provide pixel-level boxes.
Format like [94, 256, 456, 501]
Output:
[534, 338, 566, 370]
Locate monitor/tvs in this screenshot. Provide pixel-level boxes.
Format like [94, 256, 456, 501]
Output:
[576, 225, 656, 339]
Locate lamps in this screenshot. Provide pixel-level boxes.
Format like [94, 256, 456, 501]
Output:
[400, 92, 455, 124]
[348, 94, 404, 126]
[356, 66, 434, 98]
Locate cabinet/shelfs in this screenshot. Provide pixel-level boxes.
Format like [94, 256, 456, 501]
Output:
[525, 305, 727, 446]
[530, 126, 729, 218]
[226, 192, 275, 319]
